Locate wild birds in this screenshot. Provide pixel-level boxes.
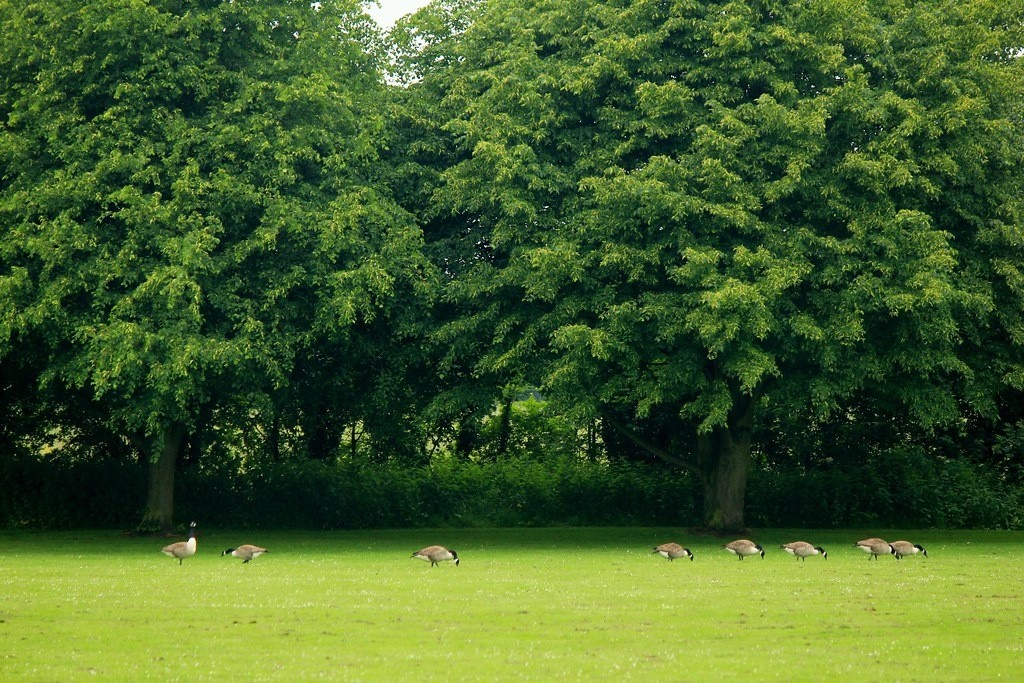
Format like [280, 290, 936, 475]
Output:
[782, 541, 828, 562]
[720, 539, 765, 561]
[410, 545, 459, 567]
[652, 543, 694, 561]
[220, 544, 269, 563]
[857, 537, 928, 562]
[162, 520, 197, 565]
